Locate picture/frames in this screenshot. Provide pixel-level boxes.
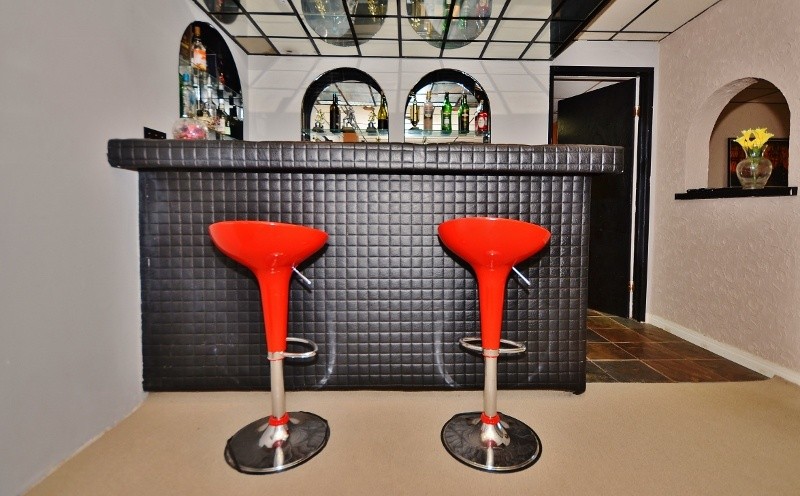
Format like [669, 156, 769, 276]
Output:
[727, 136, 789, 187]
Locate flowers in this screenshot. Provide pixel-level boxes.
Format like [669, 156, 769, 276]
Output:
[733, 126, 774, 158]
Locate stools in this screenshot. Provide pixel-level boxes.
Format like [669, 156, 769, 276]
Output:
[208, 221, 330, 476]
[437, 216, 551, 471]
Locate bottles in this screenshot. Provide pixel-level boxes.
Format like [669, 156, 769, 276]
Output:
[474, 100, 489, 136]
[442, 92, 452, 134]
[457, 94, 470, 134]
[181, 73, 196, 118]
[442, 0, 489, 32]
[378, 97, 388, 130]
[330, 93, 340, 132]
[190, 27, 207, 74]
[424, 91, 434, 119]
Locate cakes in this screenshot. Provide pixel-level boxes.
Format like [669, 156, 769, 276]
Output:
[175, 124, 206, 140]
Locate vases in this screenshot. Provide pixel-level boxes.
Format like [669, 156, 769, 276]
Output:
[735, 145, 773, 188]
